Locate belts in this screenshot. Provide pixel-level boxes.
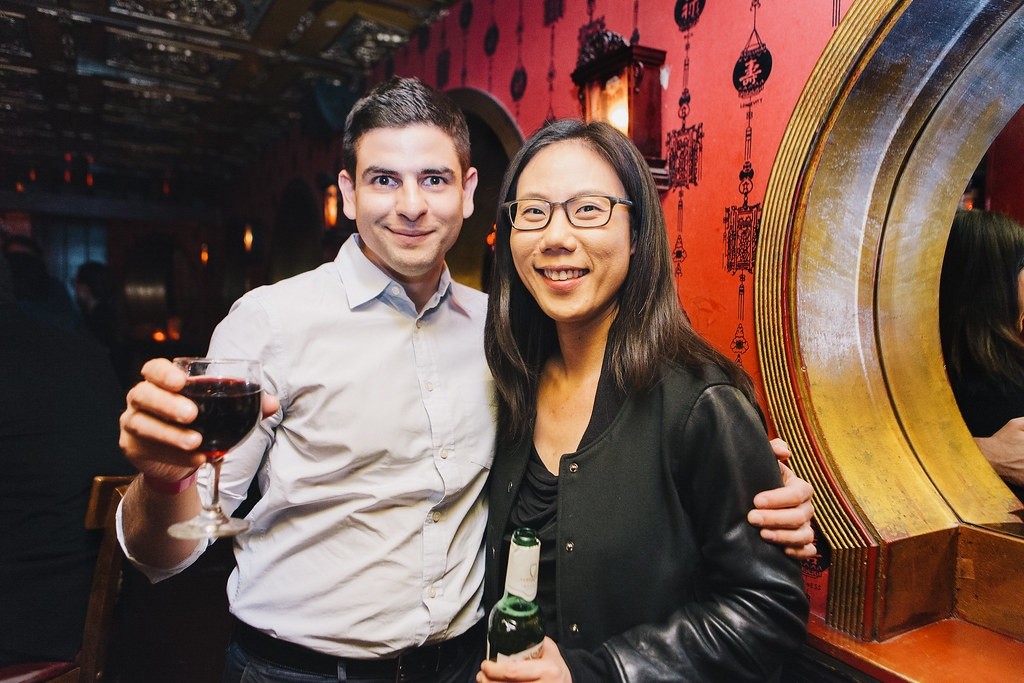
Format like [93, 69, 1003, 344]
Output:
[231, 615, 488, 682]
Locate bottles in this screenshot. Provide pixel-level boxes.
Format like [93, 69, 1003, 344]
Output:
[485, 526, 546, 661]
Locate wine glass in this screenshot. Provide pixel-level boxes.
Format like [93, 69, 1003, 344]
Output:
[166, 357, 265, 539]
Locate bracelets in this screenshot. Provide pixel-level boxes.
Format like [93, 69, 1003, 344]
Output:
[143, 468, 200, 493]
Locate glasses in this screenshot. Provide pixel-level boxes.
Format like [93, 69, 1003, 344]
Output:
[500, 194, 633, 231]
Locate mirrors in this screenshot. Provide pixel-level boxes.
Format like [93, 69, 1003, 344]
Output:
[754, 0, 1024, 645]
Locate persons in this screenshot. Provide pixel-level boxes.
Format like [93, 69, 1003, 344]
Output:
[1, 251, 118, 657]
[476, 118, 809, 683]
[118, 77, 817, 683]
[941, 210, 1024, 503]
[74, 261, 127, 362]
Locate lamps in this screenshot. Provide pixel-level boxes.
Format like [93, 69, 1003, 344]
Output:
[571, 16, 670, 192]
